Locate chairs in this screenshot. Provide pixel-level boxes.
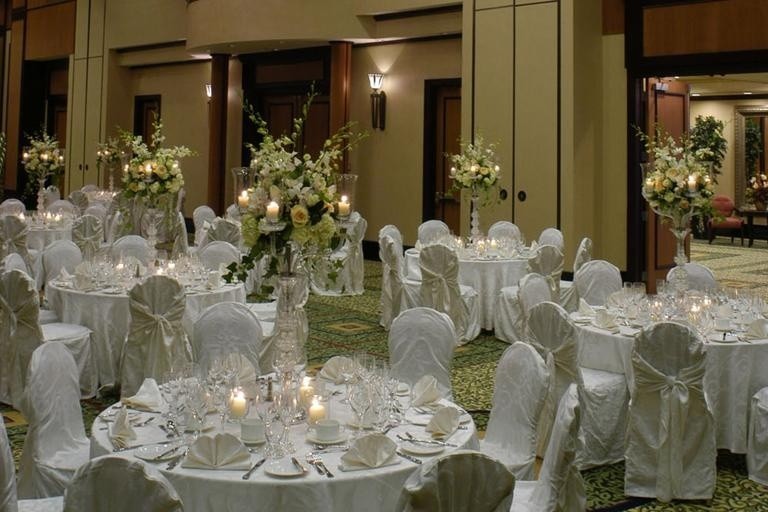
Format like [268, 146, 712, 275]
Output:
[705, 194, 744, 246]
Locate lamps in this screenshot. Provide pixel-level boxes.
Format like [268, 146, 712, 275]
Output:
[366, 72, 389, 133]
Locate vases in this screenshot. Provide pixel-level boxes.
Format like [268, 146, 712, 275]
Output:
[753, 197, 765, 212]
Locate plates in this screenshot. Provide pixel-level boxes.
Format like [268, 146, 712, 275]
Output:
[402, 438, 446, 454]
[135, 443, 186, 462]
[102, 288, 121, 295]
[307, 428, 350, 444]
[266, 458, 308, 477]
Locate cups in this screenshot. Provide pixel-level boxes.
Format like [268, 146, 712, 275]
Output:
[74, 254, 223, 292]
[241, 418, 266, 446]
[436, 233, 527, 261]
[314, 418, 345, 441]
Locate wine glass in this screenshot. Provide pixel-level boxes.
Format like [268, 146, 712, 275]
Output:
[573, 279, 766, 342]
[12, 208, 74, 229]
[166, 277, 309, 459]
[338, 348, 412, 441]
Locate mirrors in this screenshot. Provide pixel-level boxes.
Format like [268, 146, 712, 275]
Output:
[733, 103, 768, 206]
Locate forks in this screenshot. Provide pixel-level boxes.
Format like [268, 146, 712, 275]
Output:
[305, 451, 334, 479]
[99, 416, 155, 432]
[397, 430, 459, 449]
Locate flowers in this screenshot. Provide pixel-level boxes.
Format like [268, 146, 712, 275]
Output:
[221, 80, 367, 300]
[439, 129, 506, 209]
[746, 167, 768, 199]
[632, 114, 712, 237]
[97, 107, 196, 199]
[17, 129, 71, 186]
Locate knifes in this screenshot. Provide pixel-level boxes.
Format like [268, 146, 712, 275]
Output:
[242, 457, 265, 480]
